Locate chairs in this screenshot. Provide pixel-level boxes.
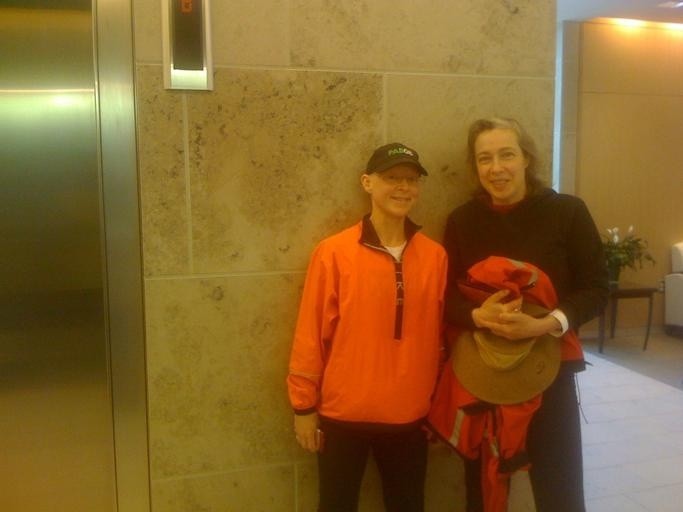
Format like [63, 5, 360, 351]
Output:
[663, 242, 682, 332]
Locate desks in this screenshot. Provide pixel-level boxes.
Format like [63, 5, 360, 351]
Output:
[594, 280, 661, 354]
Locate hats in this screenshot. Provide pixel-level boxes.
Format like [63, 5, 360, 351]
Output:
[452, 304, 560, 405]
[365, 143, 427, 176]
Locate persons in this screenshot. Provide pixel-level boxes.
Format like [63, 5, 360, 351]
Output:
[442, 116, 614, 511]
[287, 144, 447, 512]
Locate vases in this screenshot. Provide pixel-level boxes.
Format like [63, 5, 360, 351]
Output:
[606, 266, 619, 285]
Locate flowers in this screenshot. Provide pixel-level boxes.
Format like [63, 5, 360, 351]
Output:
[599, 223, 655, 281]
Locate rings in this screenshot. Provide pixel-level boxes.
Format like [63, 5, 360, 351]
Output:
[513, 307, 519, 313]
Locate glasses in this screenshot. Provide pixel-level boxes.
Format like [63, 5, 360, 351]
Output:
[376, 173, 424, 187]
